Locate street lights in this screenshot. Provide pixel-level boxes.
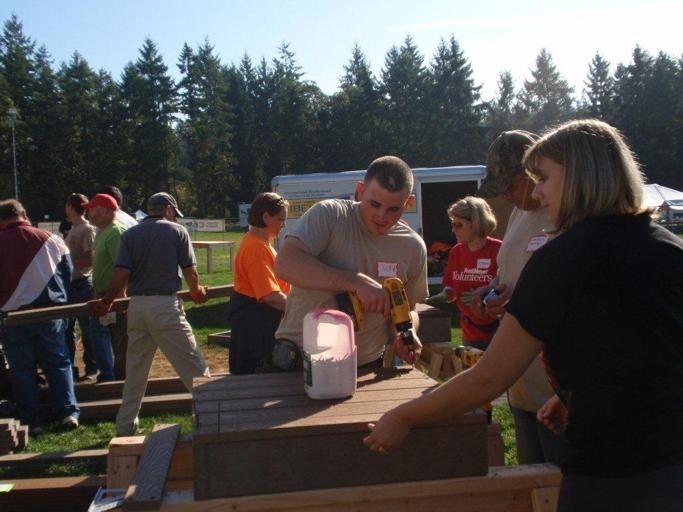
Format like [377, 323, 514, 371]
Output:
[5, 102, 23, 205]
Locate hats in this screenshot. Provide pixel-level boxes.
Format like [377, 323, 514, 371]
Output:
[79, 193, 120, 213]
[147, 191, 185, 219]
[474, 130, 541, 199]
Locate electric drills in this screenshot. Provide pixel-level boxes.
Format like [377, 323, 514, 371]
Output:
[335, 278, 418, 370]
[452, 345, 493, 424]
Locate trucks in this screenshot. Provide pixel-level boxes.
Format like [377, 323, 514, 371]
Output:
[238, 201, 256, 231]
[266, 163, 496, 299]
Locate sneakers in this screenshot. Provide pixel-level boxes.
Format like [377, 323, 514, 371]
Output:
[61, 413, 80, 430]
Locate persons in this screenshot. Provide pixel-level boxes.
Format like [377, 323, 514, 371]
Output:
[472, 130, 569, 465]
[442, 196, 503, 351]
[253, 156, 429, 373]
[0, 186, 140, 438]
[94, 192, 211, 437]
[362, 119, 683, 512]
[229, 192, 292, 375]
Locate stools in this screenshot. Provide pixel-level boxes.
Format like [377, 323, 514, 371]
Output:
[382, 338, 464, 378]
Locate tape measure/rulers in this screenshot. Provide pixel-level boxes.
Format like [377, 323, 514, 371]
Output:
[88, 486, 128, 512]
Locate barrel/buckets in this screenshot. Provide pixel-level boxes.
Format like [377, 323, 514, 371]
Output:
[302, 309, 359, 403]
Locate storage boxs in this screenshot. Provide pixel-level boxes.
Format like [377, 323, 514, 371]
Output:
[413, 298, 454, 344]
[191, 363, 489, 503]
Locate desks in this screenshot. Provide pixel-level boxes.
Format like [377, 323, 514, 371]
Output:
[191, 241, 234, 274]
